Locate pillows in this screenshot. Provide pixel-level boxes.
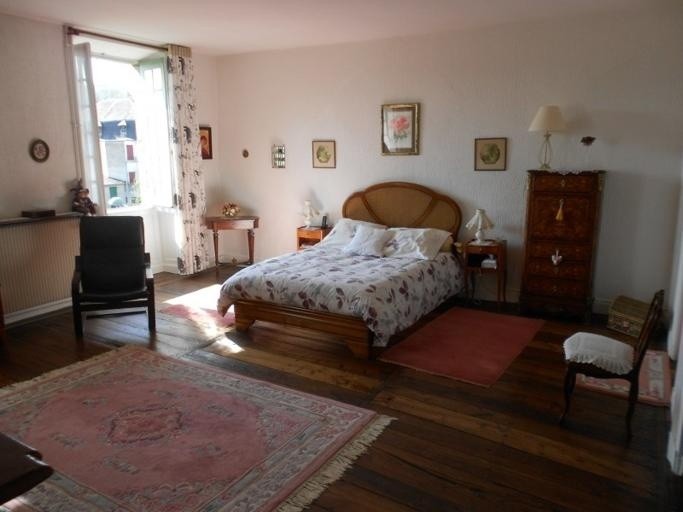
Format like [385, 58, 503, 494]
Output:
[322, 217, 453, 262]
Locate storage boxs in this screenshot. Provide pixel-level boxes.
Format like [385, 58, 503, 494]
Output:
[605, 294, 652, 340]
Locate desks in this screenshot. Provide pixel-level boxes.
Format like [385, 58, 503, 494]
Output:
[204, 216, 258, 267]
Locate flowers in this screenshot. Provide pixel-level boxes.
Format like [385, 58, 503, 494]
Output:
[222, 199, 240, 216]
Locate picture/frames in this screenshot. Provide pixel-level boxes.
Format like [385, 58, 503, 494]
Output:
[311, 140, 337, 169]
[380, 103, 420, 156]
[197, 125, 213, 160]
[473, 137, 506, 172]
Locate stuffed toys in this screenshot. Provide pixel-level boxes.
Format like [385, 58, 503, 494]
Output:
[69, 178, 97, 216]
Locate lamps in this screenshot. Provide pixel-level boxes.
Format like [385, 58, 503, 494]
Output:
[298, 201, 321, 226]
[528, 103, 570, 169]
[464, 208, 495, 243]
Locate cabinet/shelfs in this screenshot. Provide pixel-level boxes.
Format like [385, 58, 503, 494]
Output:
[516, 170, 606, 319]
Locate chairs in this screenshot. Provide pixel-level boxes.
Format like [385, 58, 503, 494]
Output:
[554, 289, 664, 441]
[69, 214, 160, 342]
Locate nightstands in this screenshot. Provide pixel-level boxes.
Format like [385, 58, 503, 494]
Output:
[295, 224, 333, 251]
[461, 237, 509, 307]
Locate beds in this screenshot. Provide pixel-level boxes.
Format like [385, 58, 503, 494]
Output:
[229, 181, 464, 360]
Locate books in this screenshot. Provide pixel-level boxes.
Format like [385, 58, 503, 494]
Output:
[21, 209, 55, 218]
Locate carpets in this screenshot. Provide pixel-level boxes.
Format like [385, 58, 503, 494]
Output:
[0, 342, 396, 510]
[377, 306, 545, 389]
[78, 280, 236, 356]
[570, 345, 672, 411]
